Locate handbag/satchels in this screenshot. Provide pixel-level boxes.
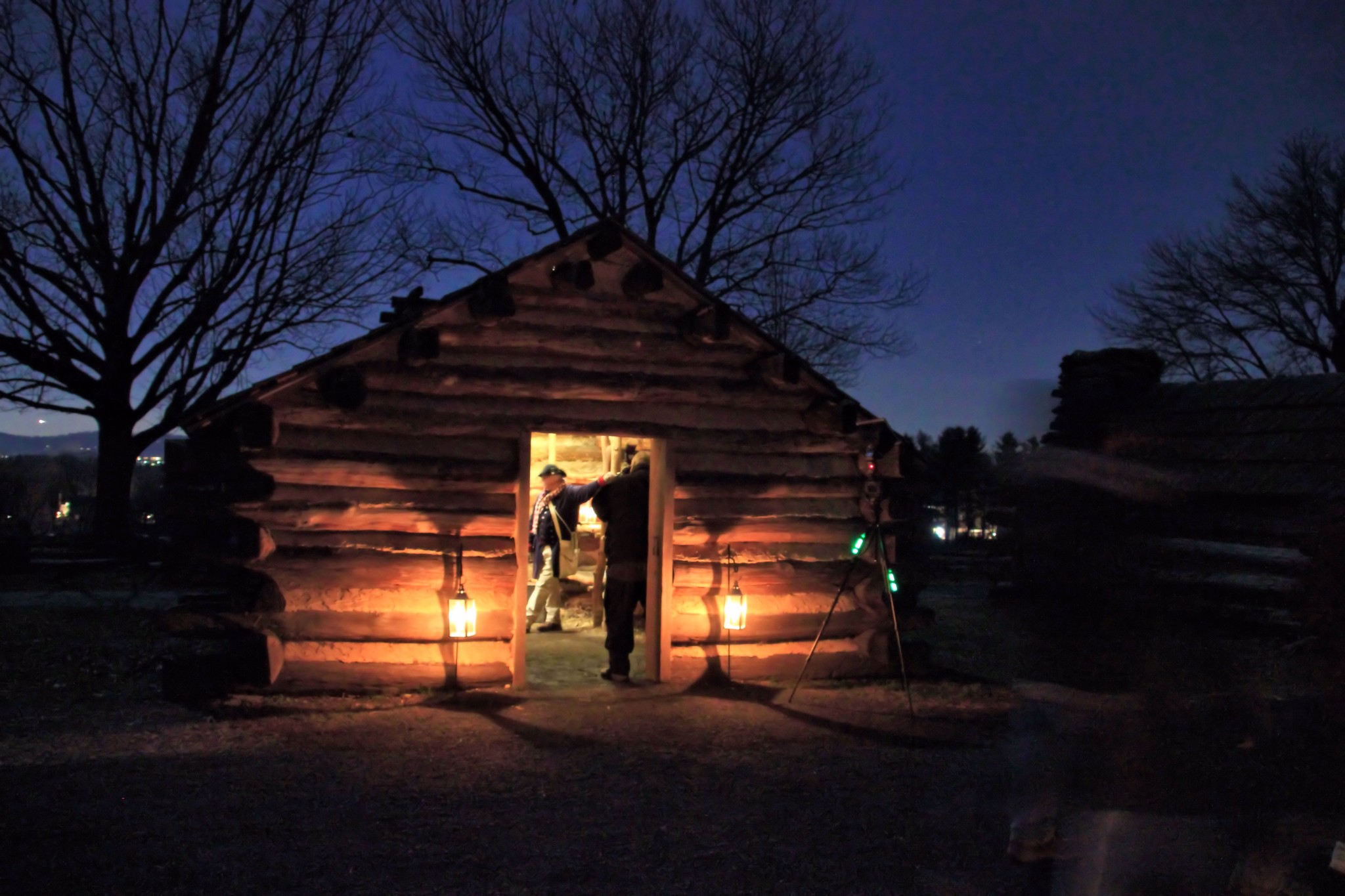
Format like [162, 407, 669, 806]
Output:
[559, 538, 579, 579]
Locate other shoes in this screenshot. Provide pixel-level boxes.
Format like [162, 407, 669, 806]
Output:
[530, 622, 562, 632]
[601, 668, 629, 681]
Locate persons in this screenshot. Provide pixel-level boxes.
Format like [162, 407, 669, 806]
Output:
[593, 450, 654, 681]
[525, 465, 620, 634]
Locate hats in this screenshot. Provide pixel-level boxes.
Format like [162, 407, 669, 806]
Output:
[538, 464, 567, 478]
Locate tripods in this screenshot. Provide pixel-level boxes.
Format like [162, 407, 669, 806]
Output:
[787, 508, 914, 715]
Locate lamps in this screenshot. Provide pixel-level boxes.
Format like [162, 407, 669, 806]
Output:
[449, 540, 475, 638]
[724, 545, 746, 629]
